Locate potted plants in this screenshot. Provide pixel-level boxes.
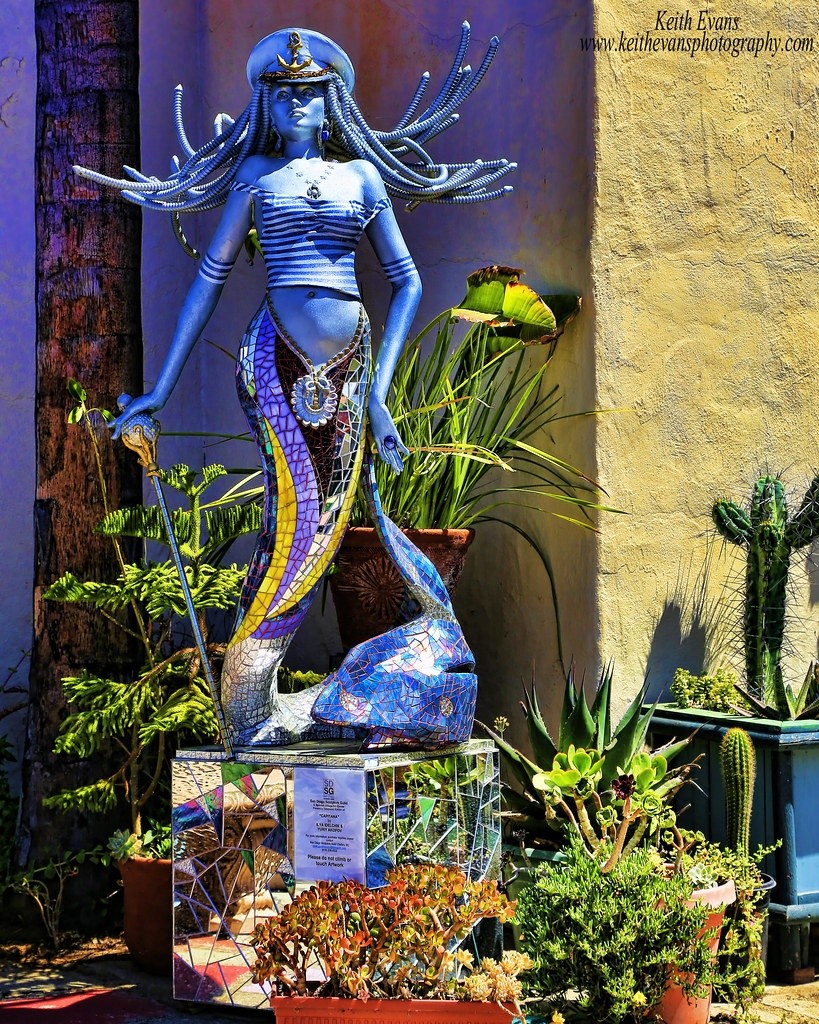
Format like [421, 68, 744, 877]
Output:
[160, 261, 633, 681]
[248, 457, 819, 1023]
[38, 459, 336, 970]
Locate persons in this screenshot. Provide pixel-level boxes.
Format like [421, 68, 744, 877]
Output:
[72, 22, 518, 473]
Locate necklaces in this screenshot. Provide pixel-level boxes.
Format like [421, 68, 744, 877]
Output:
[278, 154, 337, 196]
